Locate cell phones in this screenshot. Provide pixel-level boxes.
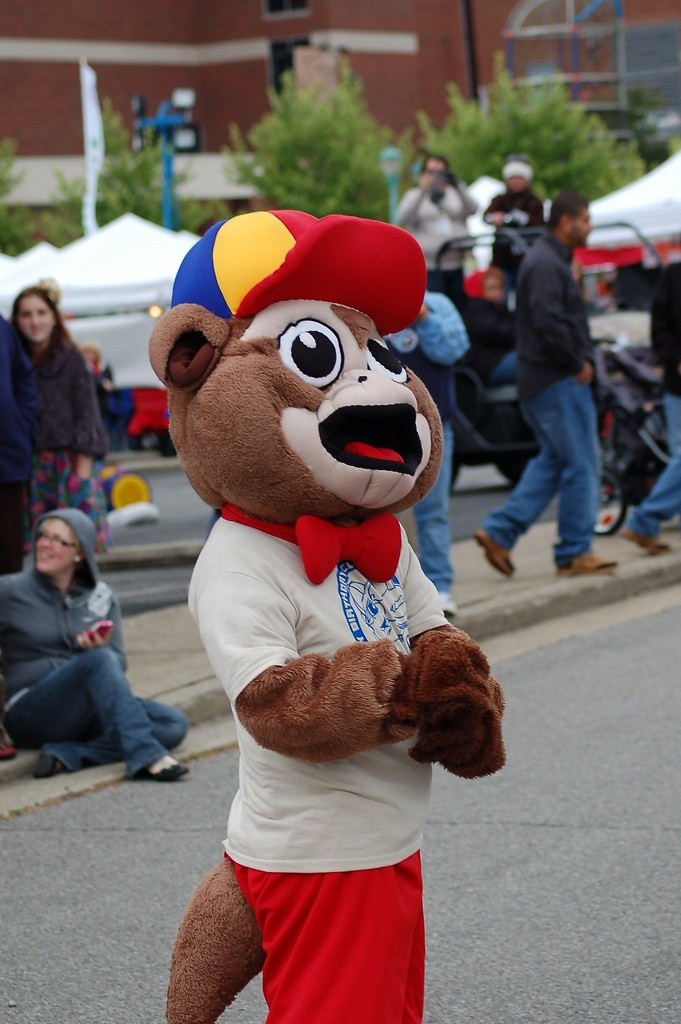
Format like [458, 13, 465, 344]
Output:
[87, 620, 113, 641]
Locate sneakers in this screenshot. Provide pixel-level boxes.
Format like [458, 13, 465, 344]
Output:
[558, 552, 617, 571]
[474, 529, 514, 574]
[622, 525, 668, 550]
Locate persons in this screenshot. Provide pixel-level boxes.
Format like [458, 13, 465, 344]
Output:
[622, 261, 681, 554]
[81, 343, 124, 424]
[0, 286, 40, 576]
[395, 153, 486, 313]
[483, 151, 546, 288]
[460, 263, 517, 373]
[473, 188, 619, 580]
[0, 508, 190, 782]
[10, 287, 113, 560]
[378, 290, 471, 619]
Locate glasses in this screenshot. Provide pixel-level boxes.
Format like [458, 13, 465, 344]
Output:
[38, 529, 80, 549]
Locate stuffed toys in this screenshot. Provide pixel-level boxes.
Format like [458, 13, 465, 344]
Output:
[148, 207, 509, 1024]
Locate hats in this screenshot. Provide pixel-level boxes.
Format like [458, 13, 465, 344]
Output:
[502, 154, 534, 181]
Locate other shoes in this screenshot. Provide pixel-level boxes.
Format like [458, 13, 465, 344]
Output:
[33, 751, 61, 776]
[439, 590, 456, 615]
[143, 754, 189, 780]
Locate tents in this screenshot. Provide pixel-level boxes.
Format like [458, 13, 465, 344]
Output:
[0, 208, 203, 392]
[570, 149, 681, 274]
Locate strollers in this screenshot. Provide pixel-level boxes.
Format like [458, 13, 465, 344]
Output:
[592, 344, 681, 537]
